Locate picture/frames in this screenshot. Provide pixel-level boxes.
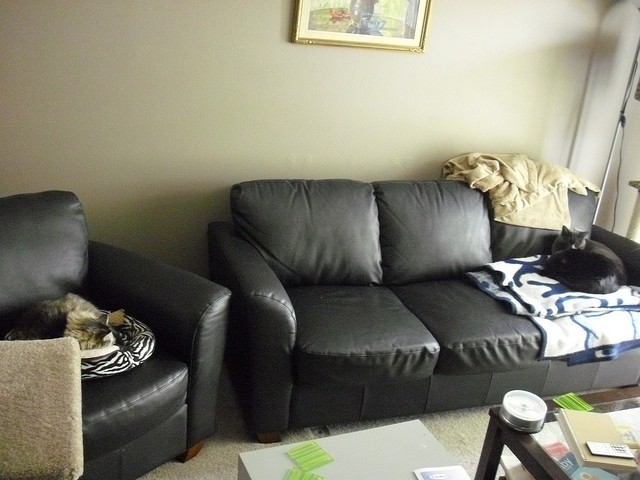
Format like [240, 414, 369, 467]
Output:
[289, 1, 438, 53]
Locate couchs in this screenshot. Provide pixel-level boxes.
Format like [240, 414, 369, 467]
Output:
[203, 177, 640, 445]
[0, 187, 233, 480]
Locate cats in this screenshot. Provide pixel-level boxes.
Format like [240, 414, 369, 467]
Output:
[15, 292, 125, 351]
[543, 225, 628, 294]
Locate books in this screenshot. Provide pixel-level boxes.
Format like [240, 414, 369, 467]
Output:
[556, 453, 620, 480]
[551, 408, 637, 471]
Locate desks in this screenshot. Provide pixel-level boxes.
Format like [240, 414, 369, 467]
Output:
[231, 418, 471, 480]
